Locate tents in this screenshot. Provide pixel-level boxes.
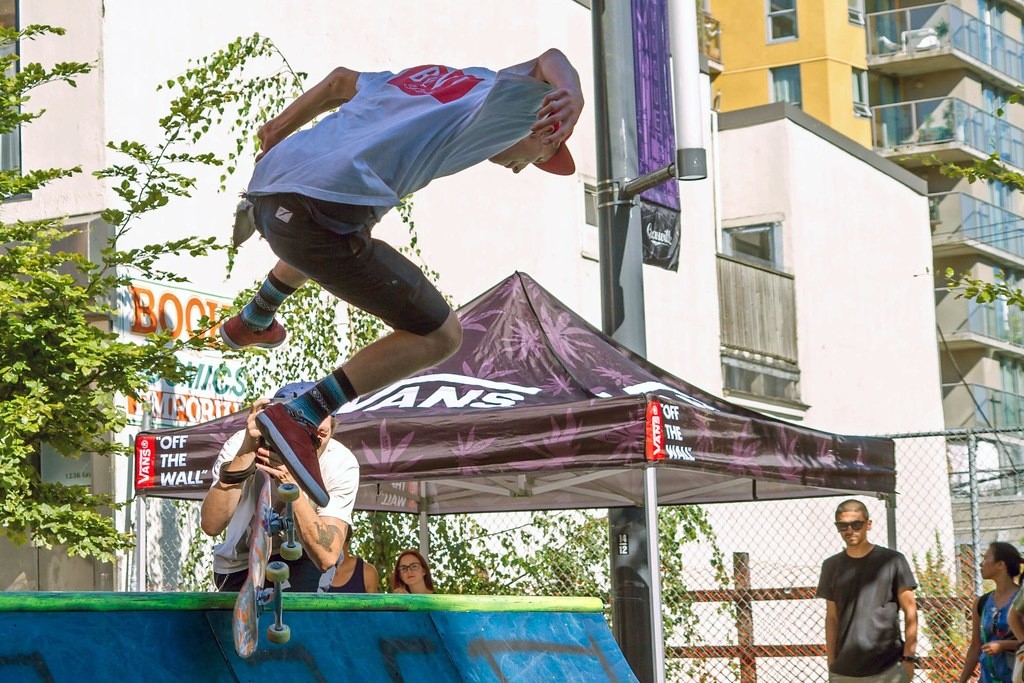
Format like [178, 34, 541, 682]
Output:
[132, 269, 902, 683]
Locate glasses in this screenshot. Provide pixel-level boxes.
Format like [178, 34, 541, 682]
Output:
[833, 519, 866, 531]
[398, 562, 421, 574]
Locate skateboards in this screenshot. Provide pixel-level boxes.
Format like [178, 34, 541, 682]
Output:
[231, 468, 304, 661]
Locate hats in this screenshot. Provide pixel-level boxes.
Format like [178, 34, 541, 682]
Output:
[533, 106, 575, 177]
[265, 382, 339, 418]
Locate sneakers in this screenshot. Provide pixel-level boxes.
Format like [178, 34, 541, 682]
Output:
[255, 402, 330, 508]
[219, 314, 290, 351]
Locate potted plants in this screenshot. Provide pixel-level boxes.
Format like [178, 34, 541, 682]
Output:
[917, 112, 948, 141]
[905, 18, 950, 53]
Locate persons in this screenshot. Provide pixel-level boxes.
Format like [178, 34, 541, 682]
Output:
[957, 540, 1023, 683]
[390, 548, 437, 596]
[817, 499, 918, 682]
[329, 522, 381, 594]
[1004, 575, 1024, 683]
[210, 46, 589, 519]
[199, 383, 361, 588]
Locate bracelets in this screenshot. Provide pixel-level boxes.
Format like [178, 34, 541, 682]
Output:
[902, 654, 920, 665]
